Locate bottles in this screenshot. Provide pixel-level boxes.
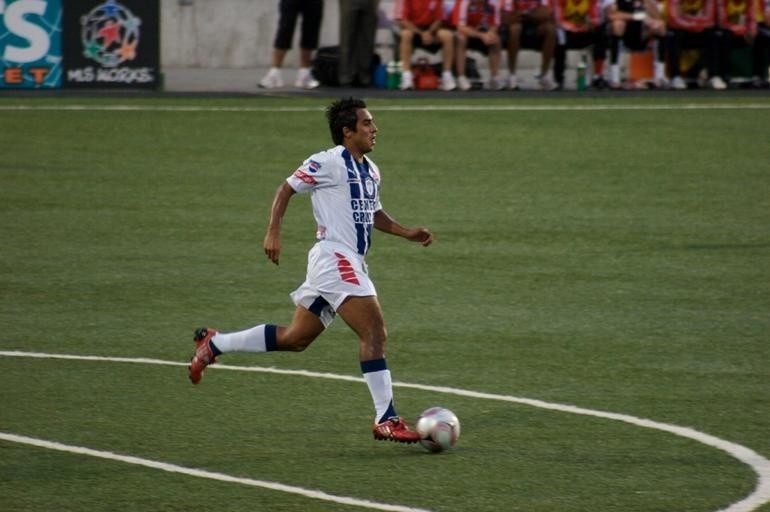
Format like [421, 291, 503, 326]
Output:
[577, 55, 592, 91]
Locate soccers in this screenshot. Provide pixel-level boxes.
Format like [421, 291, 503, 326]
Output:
[416, 407, 459, 452]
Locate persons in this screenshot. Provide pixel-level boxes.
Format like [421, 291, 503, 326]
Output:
[187, 96, 434, 445]
[256, 0, 323, 91]
[389, 1, 770, 93]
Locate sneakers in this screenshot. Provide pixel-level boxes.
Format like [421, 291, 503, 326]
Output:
[186, 324, 221, 386]
[605, 75, 729, 91]
[399, 71, 560, 91]
[254, 68, 319, 89]
[371, 416, 421, 444]
